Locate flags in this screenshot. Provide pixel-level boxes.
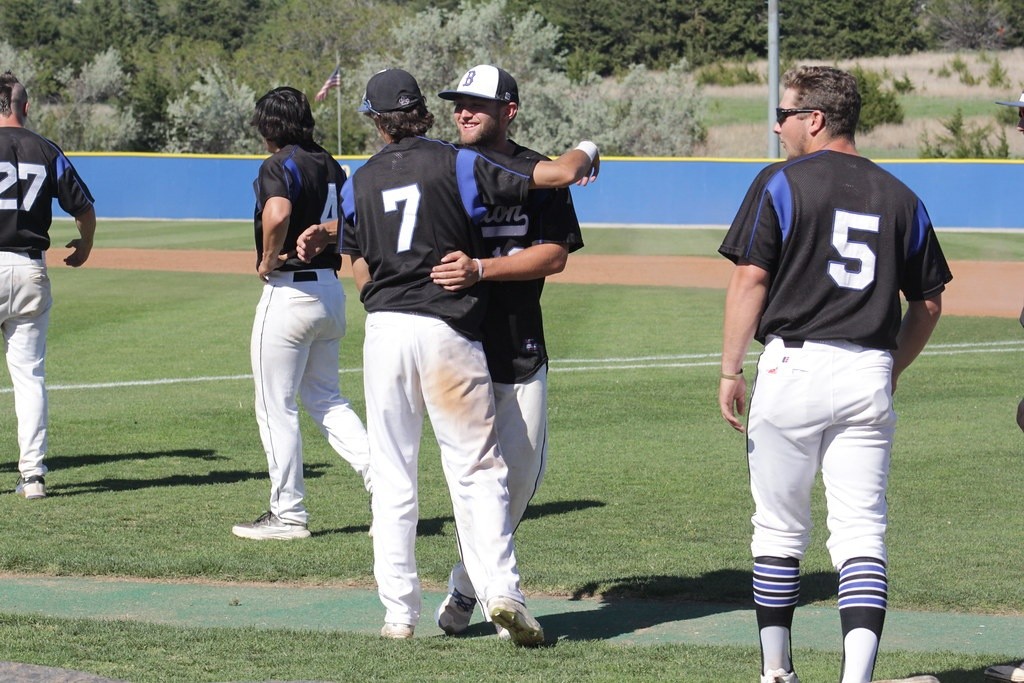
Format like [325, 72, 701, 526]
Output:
[315, 65, 340, 102]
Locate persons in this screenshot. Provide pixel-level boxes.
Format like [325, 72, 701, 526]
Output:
[0, 70, 96, 499]
[232, 86, 347, 540]
[715, 65, 953, 683]
[984, 84, 1024, 683]
[296, 65, 600, 645]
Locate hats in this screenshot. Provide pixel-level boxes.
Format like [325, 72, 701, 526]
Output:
[995, 92, 1023, 108]
[357, 69, 422, 113]
[438, 64, 520, 103]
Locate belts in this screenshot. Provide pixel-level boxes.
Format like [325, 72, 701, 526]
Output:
[293, 270, 338, 282]
[27, 249, 42, 259]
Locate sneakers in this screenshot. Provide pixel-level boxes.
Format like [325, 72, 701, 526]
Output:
[434, 575, 477, 636]
[15, 475, 46, 499]
[380, 623, 415, 639]
[761, 668, 799, 683]
[486, 595, 544, 647]
[232, 511, 311, 541]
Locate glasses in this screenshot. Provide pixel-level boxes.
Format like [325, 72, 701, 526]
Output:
[776, 107, 816, 123]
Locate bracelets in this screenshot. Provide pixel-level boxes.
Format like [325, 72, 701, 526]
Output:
[721, 368, 744, 381]
[574, 140, 598, 162]
[472, 258, 483, 281]
